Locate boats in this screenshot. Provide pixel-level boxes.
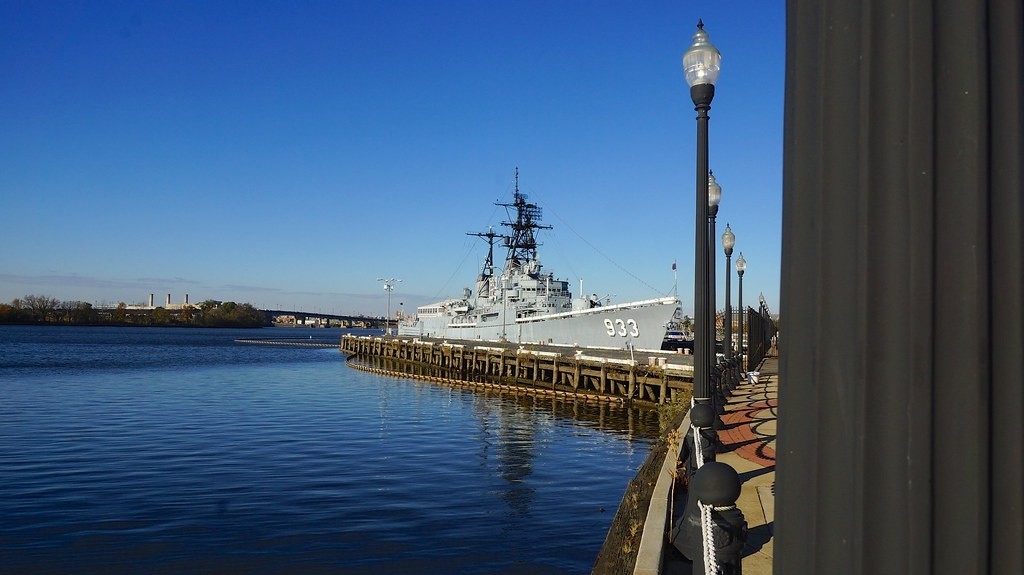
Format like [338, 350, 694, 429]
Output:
[398, 165, 678, 350]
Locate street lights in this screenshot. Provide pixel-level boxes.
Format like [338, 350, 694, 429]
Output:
[683, 16, 722, 480]
[758, 292, 773, 358]
[706, 168, 726, 431]
[735, 252, 749, 380]
[377, 277, 404, 336]
[721, 221, 733, 399]
